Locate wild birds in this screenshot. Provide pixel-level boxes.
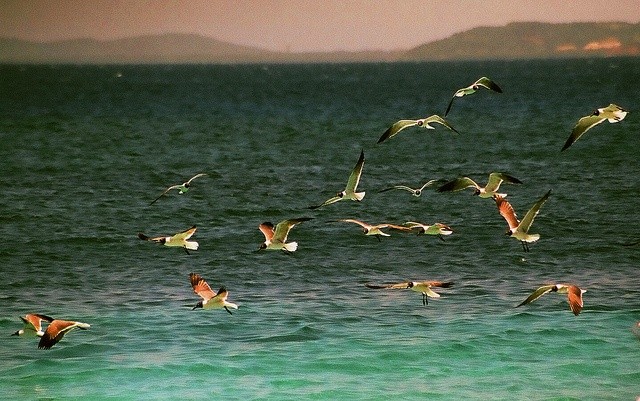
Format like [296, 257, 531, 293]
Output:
[378, 177, 449, 198]
[337, 218, 411, 237]
[148, 174, 212, 206]
[190, 273, 238, 315]
[514, 283, 587, 316]
[256, 218, 315, 255]
[34, 313, 90, 350]
[560, 104, 630, 153]
[376, 115, 462, 144]
[401, 222, 454, 243]
[138, 225, 199, 255]
[304, 149, 366, 209]
[10, 314, 44, 338]
[491, 190, 553, 252]
[445, 77, 503, 117]
[366, 281, 455, 307]
[435, 172, 523, 198]
